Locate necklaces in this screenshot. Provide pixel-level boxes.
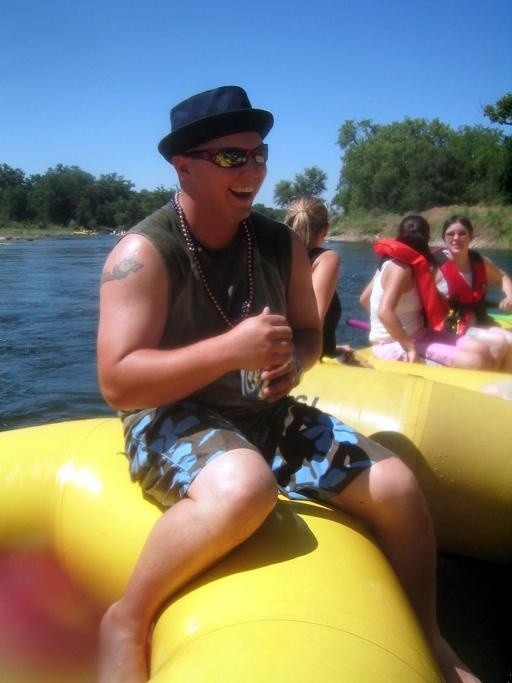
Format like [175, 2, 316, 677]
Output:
[173, 189, 252, 330]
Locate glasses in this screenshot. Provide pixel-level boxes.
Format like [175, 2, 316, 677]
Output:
[183, 144, 269, 168]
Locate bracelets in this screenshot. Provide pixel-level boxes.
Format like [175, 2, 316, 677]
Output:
[292, 352, 305, 388]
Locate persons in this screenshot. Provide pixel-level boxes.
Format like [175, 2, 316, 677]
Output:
[359, 216, 488, 369]
[429, 215, 511, 372]
[90, 84, 486, 683]
[283, 197, 368, 366]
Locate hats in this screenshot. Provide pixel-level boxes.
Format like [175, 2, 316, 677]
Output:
[157, 85, 274, 165]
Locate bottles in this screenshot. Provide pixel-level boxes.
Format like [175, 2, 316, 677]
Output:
[444, 302, 457, 334]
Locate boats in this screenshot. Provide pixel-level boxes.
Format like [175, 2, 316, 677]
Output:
[268, 310, 511, 563]
[1, 413, 434, 683]
